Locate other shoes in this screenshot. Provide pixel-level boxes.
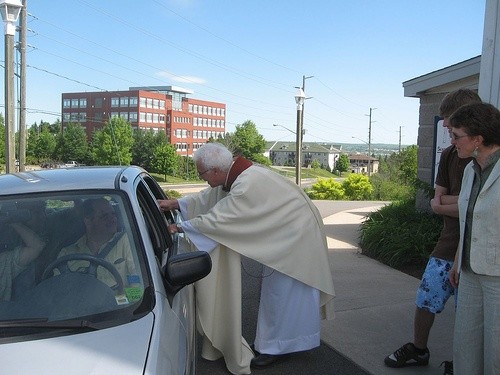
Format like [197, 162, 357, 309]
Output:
[251, 354, 288, 369]
[250, 343, 259, 356]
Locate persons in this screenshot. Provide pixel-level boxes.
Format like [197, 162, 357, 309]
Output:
[384, 88, 482, 375]
[154, 142, 336, 375]
[447, 102, 500, 375]
[0, 222, 47, 303]
[52, 199, 140, 296]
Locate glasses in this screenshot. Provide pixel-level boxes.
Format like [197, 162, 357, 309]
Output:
[197, 168, 209, 180]
[453, 133, 470, 141]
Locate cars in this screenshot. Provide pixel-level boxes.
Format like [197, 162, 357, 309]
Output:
[66, 161, 78, 166]
[0, 165, 212, 375]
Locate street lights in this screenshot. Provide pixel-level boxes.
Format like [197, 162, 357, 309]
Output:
[273, 124, 303, 186]
[293, 85, 306, 185]
[0, 0, 24, 173]
[352, 136, 370, 179]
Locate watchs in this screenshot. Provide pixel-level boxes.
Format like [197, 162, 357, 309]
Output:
[175, 223, 182, 233]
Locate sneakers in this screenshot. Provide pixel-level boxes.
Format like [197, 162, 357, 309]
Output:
[440, 360, 453, 375]
[384, 343, 430, 367]
